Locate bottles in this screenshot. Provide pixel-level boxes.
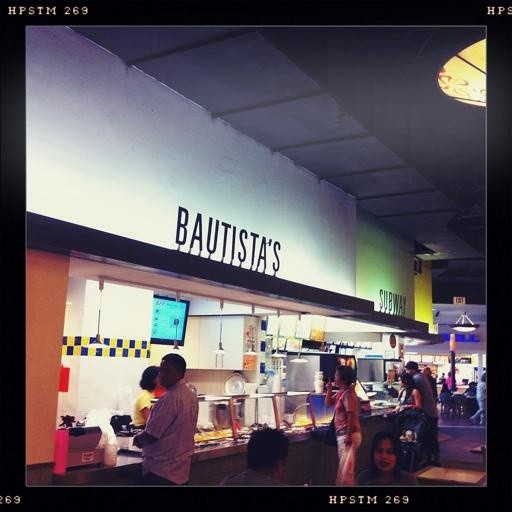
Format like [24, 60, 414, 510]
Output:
[313, 370, 324, 394]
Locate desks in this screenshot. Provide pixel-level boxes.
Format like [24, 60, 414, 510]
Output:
[469, 445, 487, 465]
[414, 466, 487, 486]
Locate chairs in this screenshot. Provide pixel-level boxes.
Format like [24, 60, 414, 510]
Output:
[438, 393, 478, 417]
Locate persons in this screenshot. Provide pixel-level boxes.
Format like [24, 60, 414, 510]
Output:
[351, 429, 419, 483]
[136, 364, 159, 428]
[394, 361, 486, 473]
[133, 353, 199, 484]
[324, 365, 362, 484]
[218, 428, 292, 487]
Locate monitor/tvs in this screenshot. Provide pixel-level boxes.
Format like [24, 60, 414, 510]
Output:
[150, 294, 191, 346]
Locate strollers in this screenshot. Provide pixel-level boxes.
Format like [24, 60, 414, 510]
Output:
[385, 407, 433, 472]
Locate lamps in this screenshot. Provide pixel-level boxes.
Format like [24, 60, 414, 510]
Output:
[84, 274, 308, 363]
[438, 39, 488, 108]
[448, 311, 480, 332]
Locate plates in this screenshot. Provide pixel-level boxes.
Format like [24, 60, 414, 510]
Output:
[222, 374, 246, 395]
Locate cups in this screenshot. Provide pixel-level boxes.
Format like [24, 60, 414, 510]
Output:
[104, 442, 118, 467]
[244, 383, 256, 394]
